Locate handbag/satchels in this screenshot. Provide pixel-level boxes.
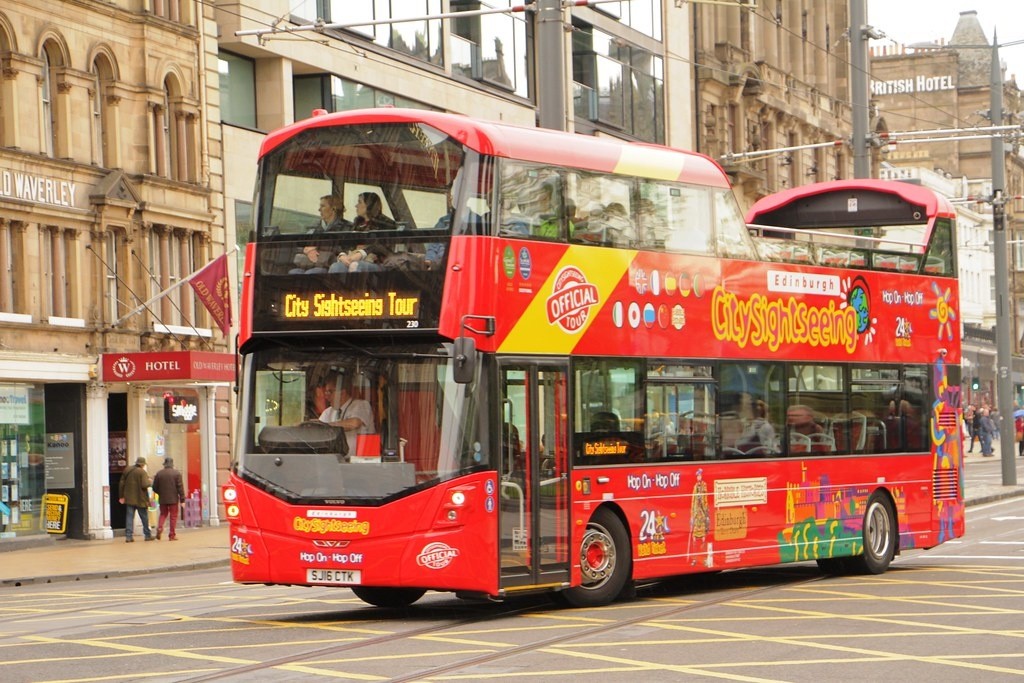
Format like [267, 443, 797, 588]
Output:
[1015, 431, 1023, 441]
[180, 506, 184, 520]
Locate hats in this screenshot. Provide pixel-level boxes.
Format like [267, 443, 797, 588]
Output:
[162, 458, 174, 467]
[135, 457, 147, 464]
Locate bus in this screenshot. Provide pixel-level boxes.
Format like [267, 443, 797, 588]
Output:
[221, 105, 970, 610]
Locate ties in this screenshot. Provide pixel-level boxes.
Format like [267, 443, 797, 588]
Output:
[336, 408, 342, 420]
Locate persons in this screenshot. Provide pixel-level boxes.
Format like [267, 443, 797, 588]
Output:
[152, 456, 186, 540]
[118, 457, 156, 543]
[294, 370, 376, 457]
[730, 391, 824, 454]
[962, 403, 1024, 457]
[287, 180, 481, 274]
[480, 182, 661, 253]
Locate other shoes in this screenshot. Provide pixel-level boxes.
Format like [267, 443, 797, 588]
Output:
[169, 536, 178, 541]
[968, 451, 972, 453]
[979, 450, 983, 453]
[983, 454, 994, 457]
[156, 528, 162, 540]
[126, 538, 134, 542]
[145, 536, 155, 541]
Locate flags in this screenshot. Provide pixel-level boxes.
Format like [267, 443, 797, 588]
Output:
[187, 252, 233, 339]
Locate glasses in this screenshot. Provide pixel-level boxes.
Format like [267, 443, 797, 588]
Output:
[324, 387, 345, 395]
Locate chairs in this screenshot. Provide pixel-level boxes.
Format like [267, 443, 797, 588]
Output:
[572, 217, 945, 277]
[651, 412, 928, 457]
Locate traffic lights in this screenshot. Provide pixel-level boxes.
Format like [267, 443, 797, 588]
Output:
[972, 377, 981, 391]
